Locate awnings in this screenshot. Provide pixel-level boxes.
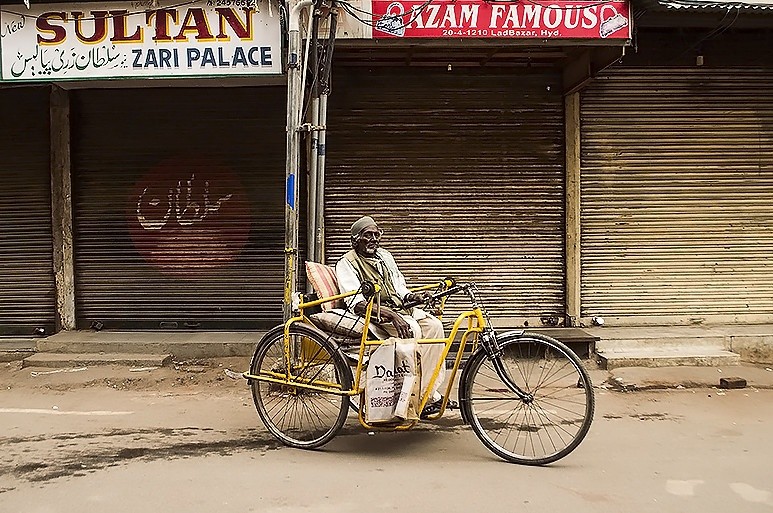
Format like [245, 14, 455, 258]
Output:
[641, 1, 773, 15]
[0, 0, 289, 86]
[300, 0, 632, 95]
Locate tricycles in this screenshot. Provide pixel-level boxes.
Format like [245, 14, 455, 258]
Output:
[244, 262, 596, 467]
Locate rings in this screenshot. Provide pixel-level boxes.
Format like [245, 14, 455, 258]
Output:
[409, 329, 412, 332]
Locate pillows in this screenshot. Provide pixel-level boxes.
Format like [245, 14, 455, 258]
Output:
[305, 261, 347, 311]
[309, 309, 365, 338]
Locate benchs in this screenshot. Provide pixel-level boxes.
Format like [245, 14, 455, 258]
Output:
[301, 293, 379, 367]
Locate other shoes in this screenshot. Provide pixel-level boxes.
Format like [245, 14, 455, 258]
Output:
[431, 395, 458, 408]
[421, 405, 440, 415]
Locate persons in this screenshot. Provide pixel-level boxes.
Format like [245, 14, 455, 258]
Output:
[335, 217, 457, 408]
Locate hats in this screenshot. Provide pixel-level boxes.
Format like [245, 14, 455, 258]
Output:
[350, 216, 377, 235]
